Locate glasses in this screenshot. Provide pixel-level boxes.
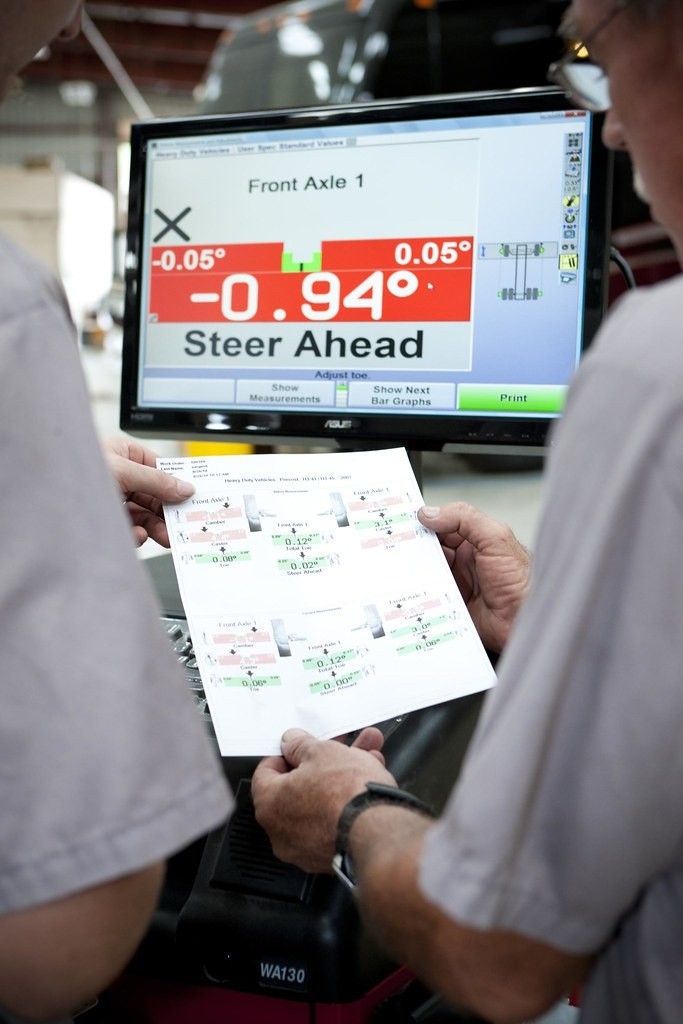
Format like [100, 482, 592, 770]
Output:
[548, 1, 632, 113]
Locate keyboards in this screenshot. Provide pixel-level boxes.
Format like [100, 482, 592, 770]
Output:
[159, 615, 216, 736]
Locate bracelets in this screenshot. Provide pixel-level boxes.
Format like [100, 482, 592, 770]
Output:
[332, 782, 435, 895]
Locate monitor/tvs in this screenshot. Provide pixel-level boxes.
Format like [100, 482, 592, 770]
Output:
[118, 88, 611, 498]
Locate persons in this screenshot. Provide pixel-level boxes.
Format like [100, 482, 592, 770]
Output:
[253, 1, 683, 1024]
[0, 0, 235, 1024]
